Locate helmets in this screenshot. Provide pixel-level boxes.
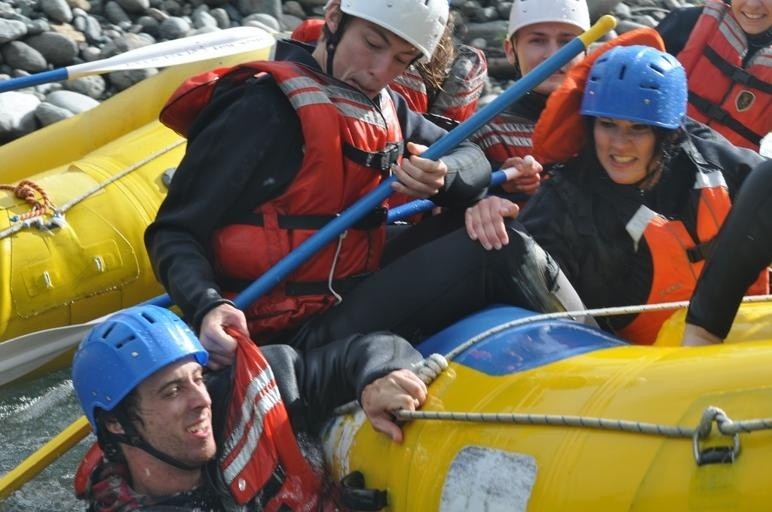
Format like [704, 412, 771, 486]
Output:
[504, 0, 593, 51]
[68, 301, 211, 435]
[576, 43, 690, 131]
[321, 0, 453, 66]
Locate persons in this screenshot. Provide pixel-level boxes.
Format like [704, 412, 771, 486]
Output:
[145, 0, 599, 348]
[644, 0, 772, 156]
[455, 41, 770, 344]
[677, 153, 771, 346]
[448, 0, 621, 207]
[71, 299, 425, 510]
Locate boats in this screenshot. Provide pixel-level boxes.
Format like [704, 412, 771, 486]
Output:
[317, 298, 772, 510]
[1, 47, 273, 378]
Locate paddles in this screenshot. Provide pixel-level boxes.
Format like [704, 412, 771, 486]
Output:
[0, 13, 617, 499]
[0, 169, 523, 387]
[0, 26, 280, 91]
